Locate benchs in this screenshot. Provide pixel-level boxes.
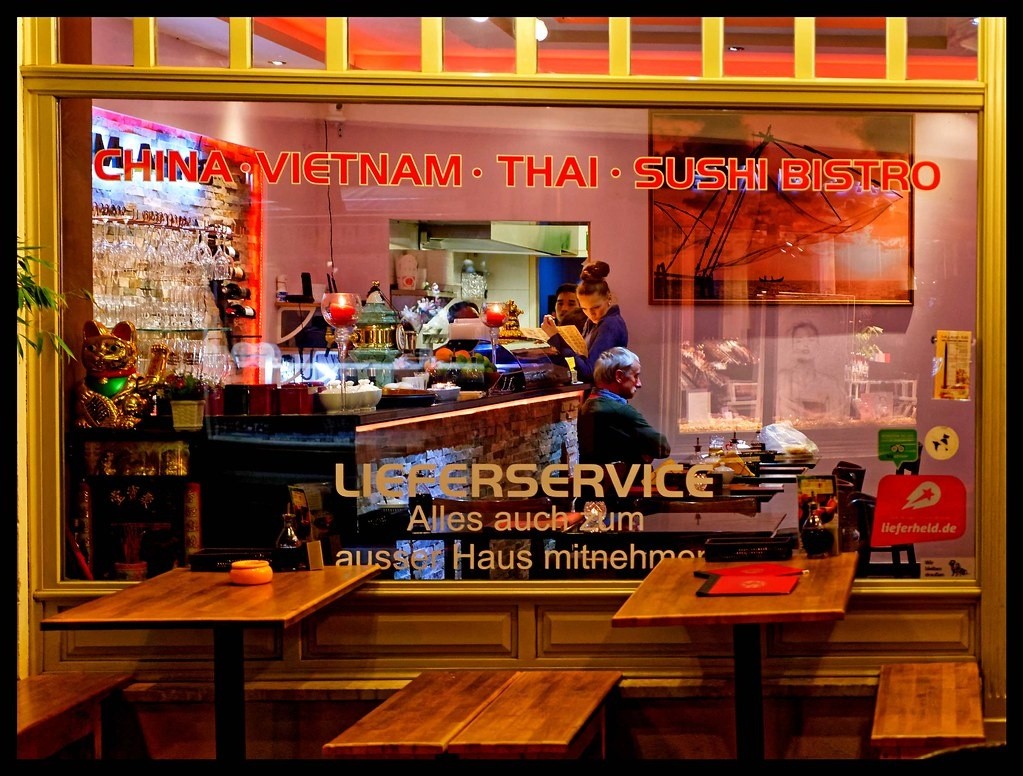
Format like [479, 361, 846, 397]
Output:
[321, 666, 519, 759]
[17, 671, 135, 759]
[449, 667, 626, 757]
[870, 662, 987, 761]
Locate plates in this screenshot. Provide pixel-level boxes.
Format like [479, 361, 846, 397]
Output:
[381, 395, 436, 408]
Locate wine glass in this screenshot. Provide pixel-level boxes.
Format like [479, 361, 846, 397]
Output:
[480, 301, 509, 373]
[320, 291, 363, 414]
[94, 211, 236, 386]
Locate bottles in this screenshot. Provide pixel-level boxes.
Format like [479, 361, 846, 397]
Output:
[396, 249, 417, 289]
[466, 356, 480, 389]
[446, 356, 461, 386]
[426, 357, 439, 385]
[730, 428, 742, 458]
[689, 438, 704, 465]
[209, 222, 256, 323]
[752, 426, 765, 447]
[800, 501, 829, 555]
[277, 513, 300, 547]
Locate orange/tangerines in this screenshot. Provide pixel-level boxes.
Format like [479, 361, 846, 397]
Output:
[424, 348, 496, 375]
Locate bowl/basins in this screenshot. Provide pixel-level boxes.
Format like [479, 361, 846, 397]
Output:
[318, 379, 383, 415]
[228, 560, 273, 585]
[714, 463, 734, 482]
[427, 386, 461, 402]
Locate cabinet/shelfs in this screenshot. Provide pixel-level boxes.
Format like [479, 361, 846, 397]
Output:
[274, 298, 325, 352]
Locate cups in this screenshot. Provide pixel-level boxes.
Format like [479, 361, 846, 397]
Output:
[417, 268, 427, 290]
[382, 371, 430, 396]
[709, 434, 725, 457]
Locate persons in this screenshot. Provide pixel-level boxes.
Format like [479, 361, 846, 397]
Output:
[431, 301, 480, 367]
[536, 260, 630, 398]
[575, 348, 671, 495]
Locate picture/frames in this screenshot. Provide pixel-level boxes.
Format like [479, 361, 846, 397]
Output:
[647, 106, 915, 308]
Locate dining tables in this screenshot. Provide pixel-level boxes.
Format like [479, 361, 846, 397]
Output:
[38, 564, 381, 762]
[398, 436, 807, 579]
[608, 551, 859, 756]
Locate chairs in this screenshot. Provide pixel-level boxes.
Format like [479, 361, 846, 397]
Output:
[826, 438, 928, 576]
